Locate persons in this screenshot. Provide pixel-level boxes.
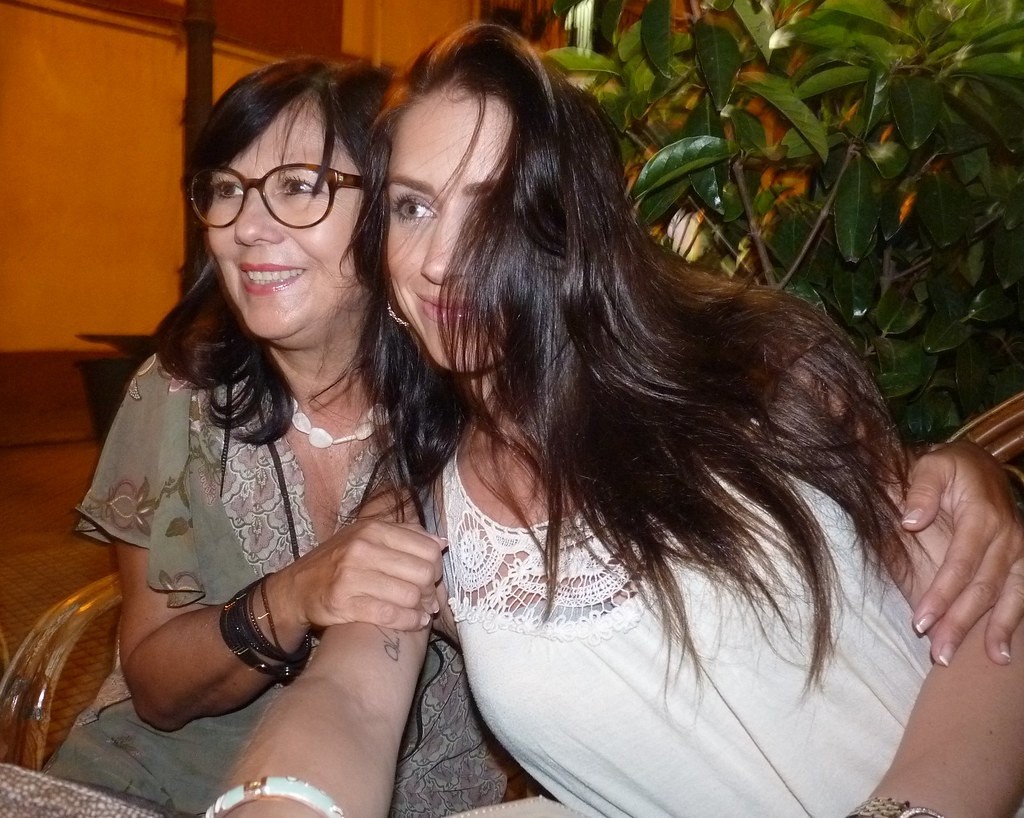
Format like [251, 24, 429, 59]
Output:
[209, 24, 1023, 818]
[44, 55, 1023, 818]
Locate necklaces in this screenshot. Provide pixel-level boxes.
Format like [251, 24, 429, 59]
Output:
[257, 405, 397, 562]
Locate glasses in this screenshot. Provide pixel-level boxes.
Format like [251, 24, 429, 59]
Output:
[187, 162, 365, 229]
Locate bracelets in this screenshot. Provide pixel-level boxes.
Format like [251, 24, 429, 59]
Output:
[206, 776, 343, 818]
[845, 798, 944, 818]
[221, 573, 310, 678]
